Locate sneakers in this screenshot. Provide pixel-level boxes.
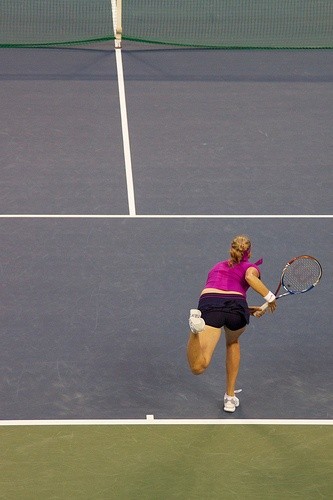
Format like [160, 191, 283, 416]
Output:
[223, 389, 241, 412]
[188, 309, 204, 334]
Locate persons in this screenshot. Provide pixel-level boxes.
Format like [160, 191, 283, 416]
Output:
[187, 236, 277, 413]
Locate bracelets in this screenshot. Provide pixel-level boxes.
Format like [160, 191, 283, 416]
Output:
[263, 291, 276, 303]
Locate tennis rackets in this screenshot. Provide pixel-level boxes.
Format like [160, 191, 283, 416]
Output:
[253, 256, 322, 318]
